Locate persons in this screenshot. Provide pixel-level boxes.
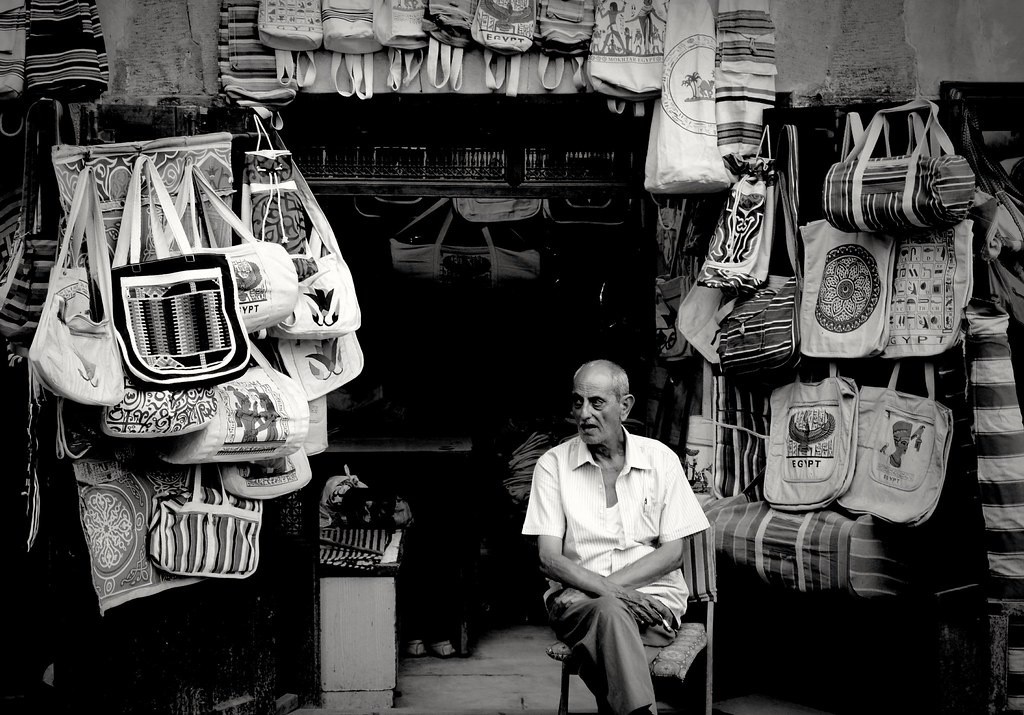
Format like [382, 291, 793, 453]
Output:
[519, 358, 713, 715]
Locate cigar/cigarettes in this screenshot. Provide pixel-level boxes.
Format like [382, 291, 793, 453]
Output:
[658, 615, 674, 634]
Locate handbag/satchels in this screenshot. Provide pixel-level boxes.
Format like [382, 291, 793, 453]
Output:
[0, 2, 1024, 608]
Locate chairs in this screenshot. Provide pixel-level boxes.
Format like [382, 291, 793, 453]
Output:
[545, 522, 716, 715]
